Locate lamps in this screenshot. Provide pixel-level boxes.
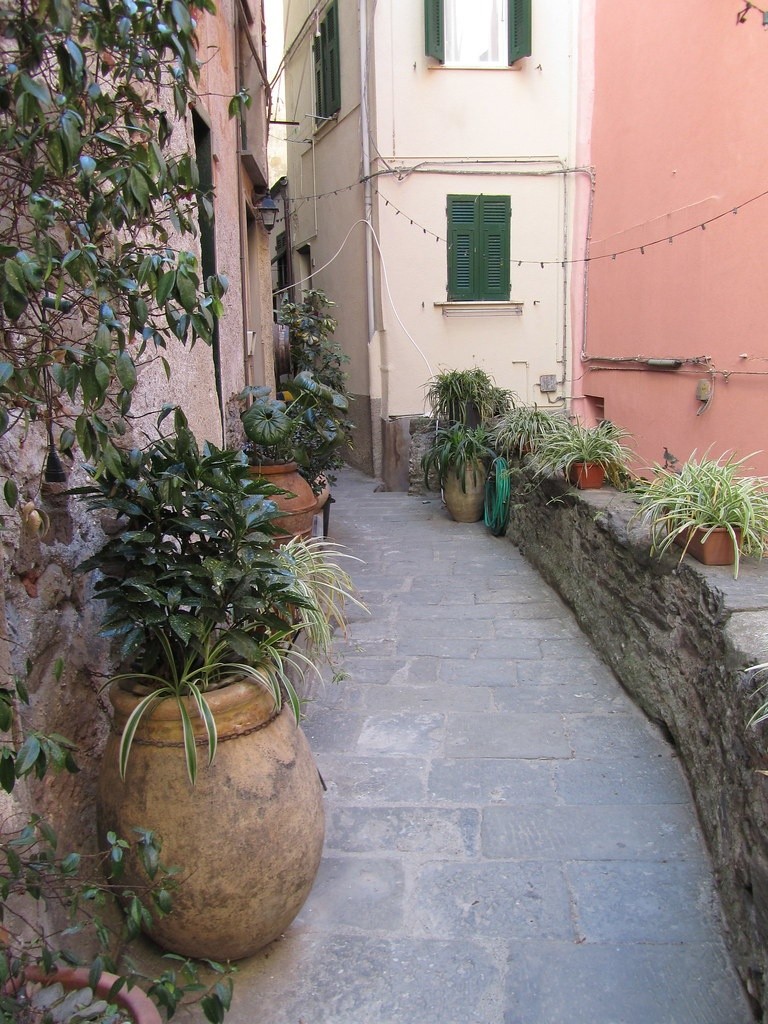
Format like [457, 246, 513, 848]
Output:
[251, 186, 280, 235]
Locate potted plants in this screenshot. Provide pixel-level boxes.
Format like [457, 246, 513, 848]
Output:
[230, 372, 349, 636]
[623, 442, 767, 579]
[47, 406, 372, 963]
[500, 415, 645, 508]
[1, 636, 237, 1024]
[416, 366, 497, 430]
[421, 422, 500, 522]
[247, 400, 356, 515]
[487, 401, 570, 461]
